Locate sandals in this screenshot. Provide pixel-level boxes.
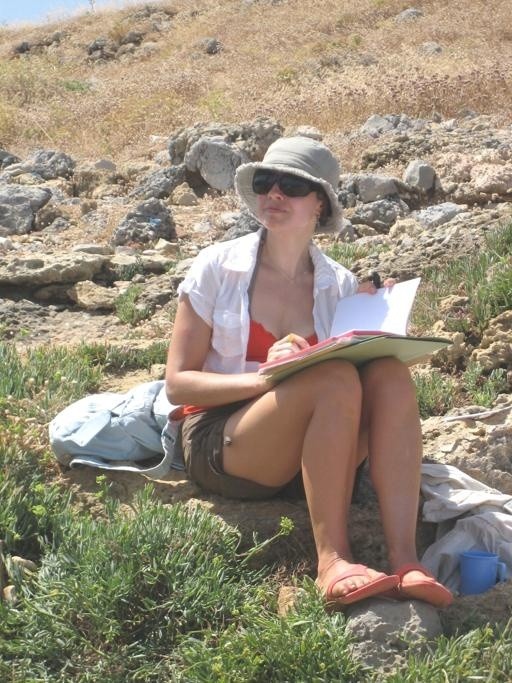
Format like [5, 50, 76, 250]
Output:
[380, 564, 454, 608]
[313, 557, 398, 612]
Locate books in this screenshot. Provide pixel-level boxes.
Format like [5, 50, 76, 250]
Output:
[255, 276, 452, 384]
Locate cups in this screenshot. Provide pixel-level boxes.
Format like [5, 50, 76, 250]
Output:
[460, 550, 507, 597]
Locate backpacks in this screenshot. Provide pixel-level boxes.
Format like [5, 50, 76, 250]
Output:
[47, 378, 186, 478]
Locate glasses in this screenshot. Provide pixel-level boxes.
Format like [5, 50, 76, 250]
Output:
[252, 169, 319, 197]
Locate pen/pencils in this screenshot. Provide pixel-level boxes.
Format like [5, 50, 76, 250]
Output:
[373, 272, 381, 289]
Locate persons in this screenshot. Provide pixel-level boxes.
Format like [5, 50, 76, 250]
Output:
[161, 134, 454, 613]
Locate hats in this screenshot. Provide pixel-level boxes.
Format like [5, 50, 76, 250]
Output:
[231, 134, 347, 237]
